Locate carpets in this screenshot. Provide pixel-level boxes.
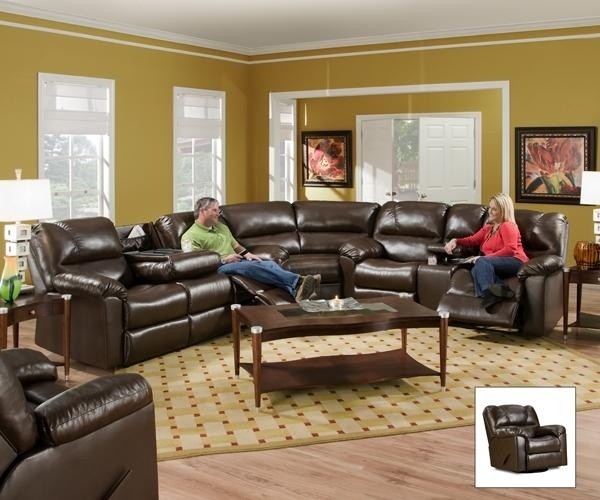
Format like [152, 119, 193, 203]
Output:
[114, 326, 600, 461]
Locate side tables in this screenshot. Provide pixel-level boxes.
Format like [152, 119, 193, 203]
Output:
[563, 266, 600, 345]
[0, 292, 73, 383]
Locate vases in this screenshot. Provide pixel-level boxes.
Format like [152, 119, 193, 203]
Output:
[0, 256, 22, 303]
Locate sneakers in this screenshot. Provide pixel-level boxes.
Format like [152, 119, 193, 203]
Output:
[481, 295, 498, 308]
[491, 283, 513, 298]
[296, 273, 321, 302]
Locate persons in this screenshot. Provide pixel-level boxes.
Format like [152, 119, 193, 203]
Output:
[444, 192, 530, 310]
[180, 197, 323, 302]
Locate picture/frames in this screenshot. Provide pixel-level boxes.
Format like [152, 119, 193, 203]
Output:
[514, 126, 596, 206]
[301, 129, 353, 189]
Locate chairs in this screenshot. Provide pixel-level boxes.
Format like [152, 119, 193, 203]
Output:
[0, 347, 158, 500]
[483, 404, 568, 473]
